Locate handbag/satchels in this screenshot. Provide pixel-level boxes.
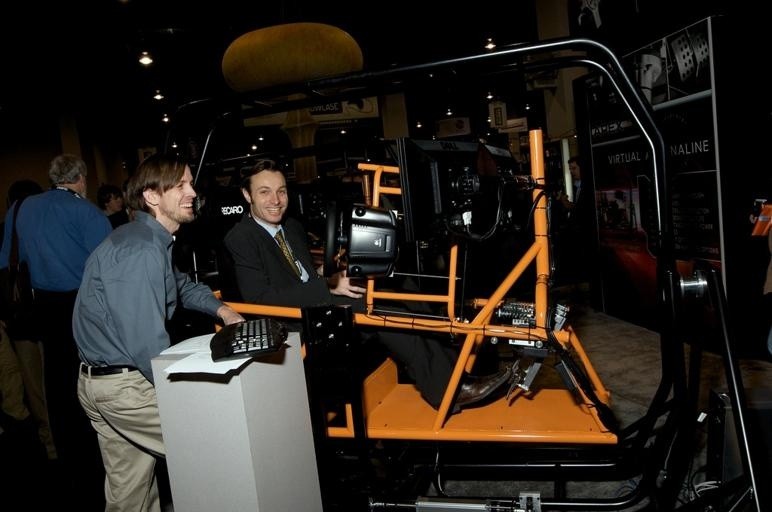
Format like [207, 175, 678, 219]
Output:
[0, 193, 41, 344]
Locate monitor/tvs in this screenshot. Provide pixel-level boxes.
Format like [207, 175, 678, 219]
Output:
[395, 136, 534, 243]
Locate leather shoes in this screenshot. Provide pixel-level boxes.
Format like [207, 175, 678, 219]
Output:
[448, 367, 514, 415]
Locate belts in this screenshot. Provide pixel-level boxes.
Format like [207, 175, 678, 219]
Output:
[81, 363, 139, 377]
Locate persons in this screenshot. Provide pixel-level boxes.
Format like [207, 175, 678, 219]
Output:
[94, 184, 126, 214]
[1, 179, 59, 463]
[1, 150, 111, 471]
[221, 155, 513, 414]
[108, 174, 133, 233]
[70, 148, 247, 511]
[567, 157, 583, 202]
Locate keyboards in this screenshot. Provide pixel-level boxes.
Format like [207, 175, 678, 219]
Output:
[210, 318, 287, 362]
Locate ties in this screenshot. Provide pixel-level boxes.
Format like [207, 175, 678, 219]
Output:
[274, 229, 301, 281]
